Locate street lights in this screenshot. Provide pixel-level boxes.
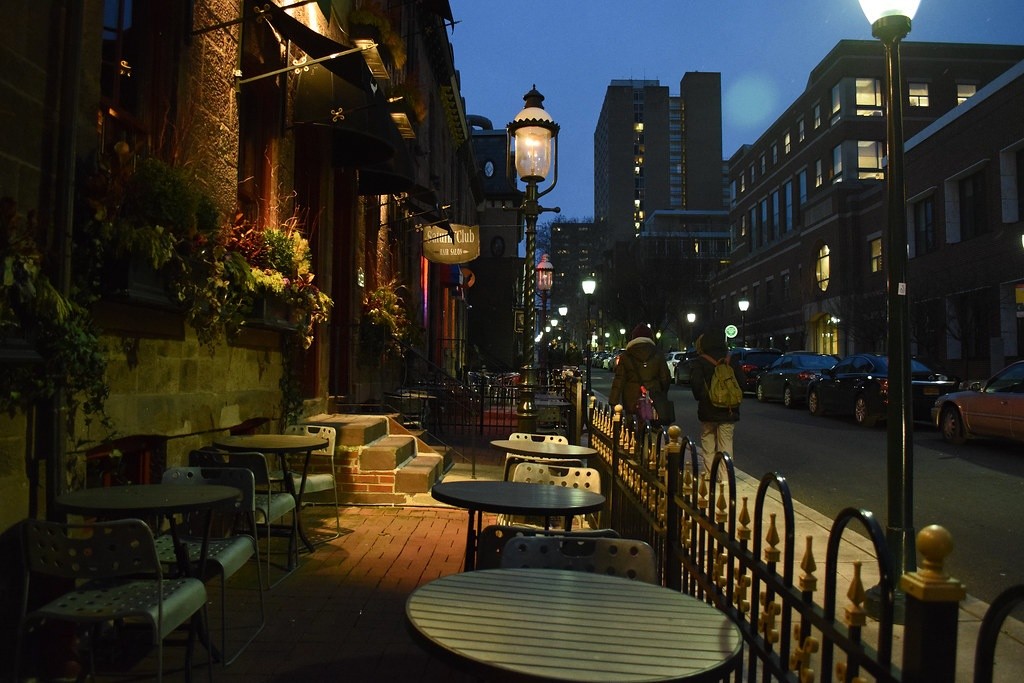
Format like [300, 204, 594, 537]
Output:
[857, 0, 922, 625]
[619, 329, 626, 348]
[506, 83, 561, 435]
[581, 274, 596, 397]
[738, 300, 749, 347]
[558, 304, 568, 354]
[535, 250, 554, 394]
[550, 317, 559, 339]
[686, 314, 696, 342]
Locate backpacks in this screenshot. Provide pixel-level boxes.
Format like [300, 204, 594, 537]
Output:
[699, 353, 743, 410]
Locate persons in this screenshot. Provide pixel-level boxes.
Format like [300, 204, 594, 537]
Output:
[690, 328, 740, 482]
[610, 325, 671, 492]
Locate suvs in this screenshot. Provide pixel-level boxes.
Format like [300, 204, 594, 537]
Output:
[727, 347, 784, 392]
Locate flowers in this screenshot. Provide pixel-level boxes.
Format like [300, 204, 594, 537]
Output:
[77, 154, 332, 432]
[359, 276, 426, 367]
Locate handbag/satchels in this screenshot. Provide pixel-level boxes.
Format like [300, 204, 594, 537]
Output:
[655, 398, 675, 426]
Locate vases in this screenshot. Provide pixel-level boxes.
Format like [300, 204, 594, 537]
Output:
[238, 292, 300, 335]
[373, 325, 392, 342]
[101, 258, 173, 309]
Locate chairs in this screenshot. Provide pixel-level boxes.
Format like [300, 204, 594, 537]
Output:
[536, 407, 567, 437]
[274, 424, 340, 545]
[154, 467, 266, 669]
[188, 449, 301, 589]
[514, 462, 601, 494]
[509, 432, 568, 461]
[21, 517, 210, 683]
[475, 526, 621, 573]
[502, 537, 657, 584]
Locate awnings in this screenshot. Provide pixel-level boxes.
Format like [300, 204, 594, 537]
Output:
[237, 0, 403, 129]
[189, 0, 453, 34]
[295, 61, 454, 246]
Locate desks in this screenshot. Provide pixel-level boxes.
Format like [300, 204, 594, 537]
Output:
[534, 396, 567, 401]
[65, 481, 241, 683]
[211, 434, 328, 571]
[431, 480, 608, 572]
[402, 568, 743, 683]
[490, 440, 598, 481]
[534, 402, 573, 428]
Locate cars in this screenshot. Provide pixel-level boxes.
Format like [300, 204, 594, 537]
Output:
[807, 353, 961, 428]
[583, 349, 625, 373]
[930, 359, 1024, 446]
[755, 351, 841, 409]
[674, 347, 699, 385]
[665, 352, 686, 384]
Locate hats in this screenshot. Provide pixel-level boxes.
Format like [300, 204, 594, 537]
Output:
[632, 323, 653, 338]
